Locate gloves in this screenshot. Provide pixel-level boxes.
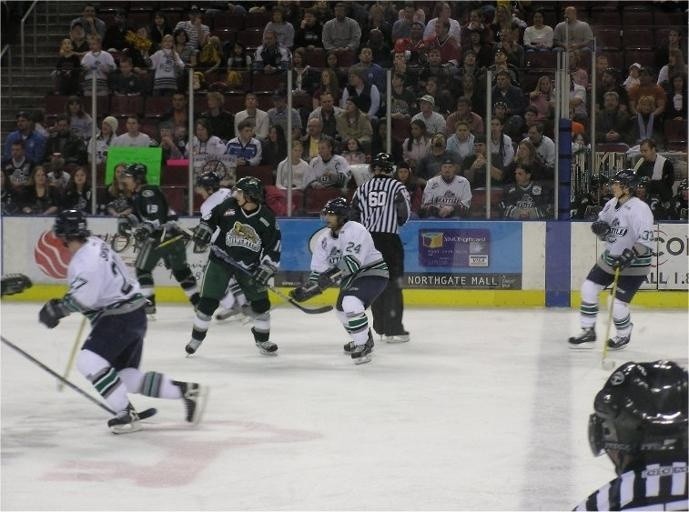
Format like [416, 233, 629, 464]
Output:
[37, 298, 71, 330]
[289, 285, 315, 303]
[590, 220, 612, 237]
[117, 215, 132, 238]
[605, 248, 635, 272]
[247, 259, 279, 286]
[191, 218, 218, 250]
[133, 227, 151, 242]
[317, 267, 340, 289]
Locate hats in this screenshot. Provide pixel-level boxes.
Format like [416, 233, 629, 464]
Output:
[102, 115, 119, 134]
[396, 160, 410, 170]
[346, 95, 360, 108]
[271, 88, 285, 100]
[440, 156, 455, 165]
[15, 110, 31, 121]
[415, 93, 436, 107]
[188, 4, 201, 15]
[493, 96, 508, 112]
[629, 62, 642, 70]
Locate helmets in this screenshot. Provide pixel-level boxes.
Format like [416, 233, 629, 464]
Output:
[322, 197, 352, 216]
[609, 169, 643, 188]
[122, 161, 148, 177]
[587, 358, 688, 457]
[233, 175, 267, 203]
[53, 208, 91, 238]
[372, 152, 395, 168]
[639, 176, 652, 191]
[678, 178, 688, 191]
[197, 172, 221, 188]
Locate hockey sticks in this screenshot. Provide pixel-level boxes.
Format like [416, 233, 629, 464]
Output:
[0, 335, 155, 421]
[171, 220, 333, 314]
[602, 264, 618, 370]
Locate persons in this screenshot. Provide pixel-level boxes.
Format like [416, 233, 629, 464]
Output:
[346, 152, 409, 337]
[184, 176, 281, 356]
[194, 170, 251, 320]
[116, 162, 201, 314]
[38, 209, 201, 427]
[565, 168, 656, 350]
[289, 196, 391, 360]
[572, 359, 689, 511]
[1, 0, 689, 220]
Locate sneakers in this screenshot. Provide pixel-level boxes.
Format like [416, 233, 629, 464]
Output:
[343, 327, 375, 360]
[254, 339, 278, 352]
[185, 333, 205, 355]
[568, 328, 598, 344]
[143, 302, 156, 315]
[373, 325, 410, 337]
[106, 403, 141, 426]
[215, 303, 253, 321]
[179, 380, 201, 423]
[607, 334, 630, 349]
